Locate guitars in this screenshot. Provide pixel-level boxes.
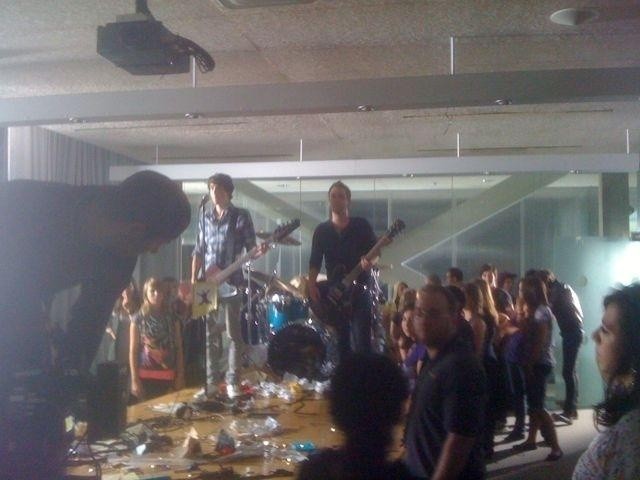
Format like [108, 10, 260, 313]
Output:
[180, 217, 299, 320]
[309, 219, 406, 325]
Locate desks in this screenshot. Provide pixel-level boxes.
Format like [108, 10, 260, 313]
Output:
[63, 375, 417, 479]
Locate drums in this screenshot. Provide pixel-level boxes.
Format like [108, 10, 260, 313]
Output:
[268, 322, 337, 383]
[266, 294, 310, 333]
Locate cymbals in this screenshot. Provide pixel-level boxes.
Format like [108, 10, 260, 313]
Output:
[255, 230, 301, 246]
[245, 269, 301, 298]
[289, 273, 326, 297]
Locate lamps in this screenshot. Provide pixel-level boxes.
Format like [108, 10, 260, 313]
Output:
[548, 6, 602, 27]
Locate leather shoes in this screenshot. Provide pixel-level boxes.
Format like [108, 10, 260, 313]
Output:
[552, 412, 572, 427]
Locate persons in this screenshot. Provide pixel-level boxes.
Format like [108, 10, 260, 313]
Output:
[571, 276, 640, 480]
[308, 181, 381, 369]
[105, 273, 286, 403]
[0, 169, 193, 480]
[190, 173, 271, 400]
[287, 262, 587, 479]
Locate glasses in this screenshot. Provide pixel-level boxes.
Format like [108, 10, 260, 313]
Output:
[411, 309, 449, 320]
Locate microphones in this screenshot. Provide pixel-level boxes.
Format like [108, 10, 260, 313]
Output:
[199, 193, 210, 208]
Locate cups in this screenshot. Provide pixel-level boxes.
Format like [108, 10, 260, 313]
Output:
[244, 439, 292, 477]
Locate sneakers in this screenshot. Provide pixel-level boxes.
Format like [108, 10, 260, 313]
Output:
[193, 388, 219, 399]
[227, 383, 248, 399]
[485, 428, 564, 461]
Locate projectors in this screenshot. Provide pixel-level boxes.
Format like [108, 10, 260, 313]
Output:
[97, 12, 190, 76]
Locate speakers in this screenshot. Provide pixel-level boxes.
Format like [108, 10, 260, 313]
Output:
[78, 361, 126, 438]
[600, 172, 630, 242]
